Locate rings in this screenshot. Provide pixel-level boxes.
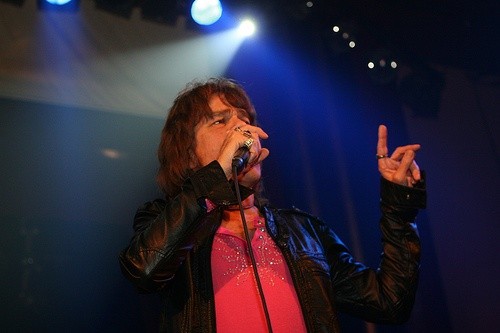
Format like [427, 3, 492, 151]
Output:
[376, 154, 388, 158]
[235, 127, 253, 149]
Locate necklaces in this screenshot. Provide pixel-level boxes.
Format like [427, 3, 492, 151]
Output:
[225, 204, 254, 211]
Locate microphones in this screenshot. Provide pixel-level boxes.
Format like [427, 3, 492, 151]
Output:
[232, 146, 251, 172]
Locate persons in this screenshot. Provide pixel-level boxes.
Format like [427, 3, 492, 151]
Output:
[118, 79, 427, 333]
